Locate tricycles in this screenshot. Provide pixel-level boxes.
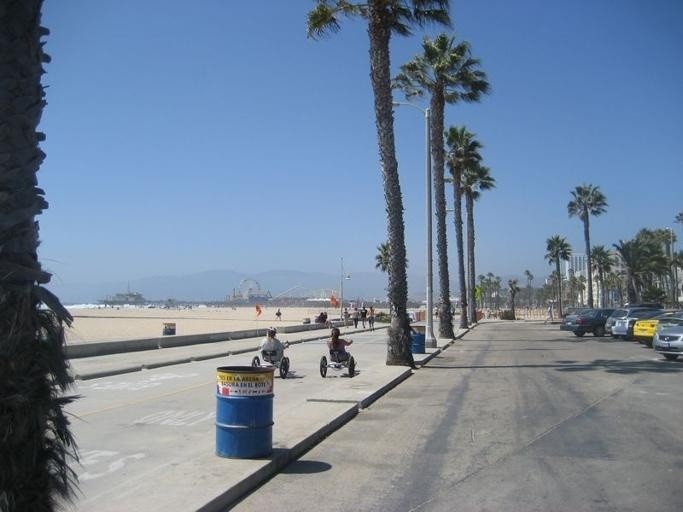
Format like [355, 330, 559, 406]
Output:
[251, 341, 290, 379]
[320, 340, 355, 377]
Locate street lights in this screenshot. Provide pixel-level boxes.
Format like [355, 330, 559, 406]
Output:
[389, 98, 437, 349]
[339, 256, 351, 318]
[444, 209, 470, 326]
[664, 225, 673, 271]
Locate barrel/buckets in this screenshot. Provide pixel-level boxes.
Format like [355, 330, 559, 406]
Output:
[303, 318, 310, 324]
[410, 334, 424, 353]
[162, 323, 175, 335]
[214, 365, 274, 458]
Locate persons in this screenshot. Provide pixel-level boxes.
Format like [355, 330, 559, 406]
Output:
[314, 305, 377, 331]
[326, 328, 353, 366]
[260, 327, 285, 363]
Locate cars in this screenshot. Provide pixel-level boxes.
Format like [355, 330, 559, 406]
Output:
[563, 304, 680, 348]
[652, 322, 682, 360]
